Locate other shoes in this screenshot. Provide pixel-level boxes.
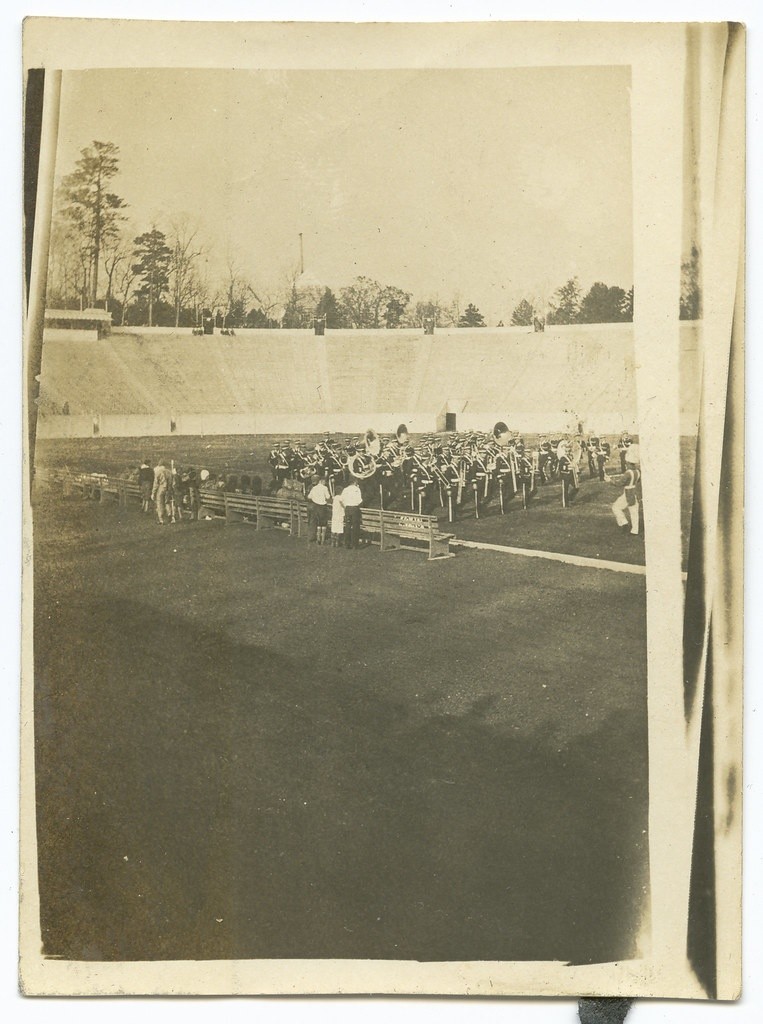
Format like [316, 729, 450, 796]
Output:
[157, 521, 165, 525]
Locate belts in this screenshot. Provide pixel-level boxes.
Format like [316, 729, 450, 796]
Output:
[274, 464, 642, 489]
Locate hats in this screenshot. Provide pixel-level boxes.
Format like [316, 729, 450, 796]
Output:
[273, 421, 642, 465]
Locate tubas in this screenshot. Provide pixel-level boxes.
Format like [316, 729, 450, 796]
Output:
[346, 427, 382, 481]
[388, 422, 410, 446]
[485, 420, 513, 450]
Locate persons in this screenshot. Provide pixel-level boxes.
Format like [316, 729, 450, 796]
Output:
[121, 431, 642, 549]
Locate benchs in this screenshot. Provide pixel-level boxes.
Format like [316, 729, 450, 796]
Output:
[33, 465, 457, 561]
